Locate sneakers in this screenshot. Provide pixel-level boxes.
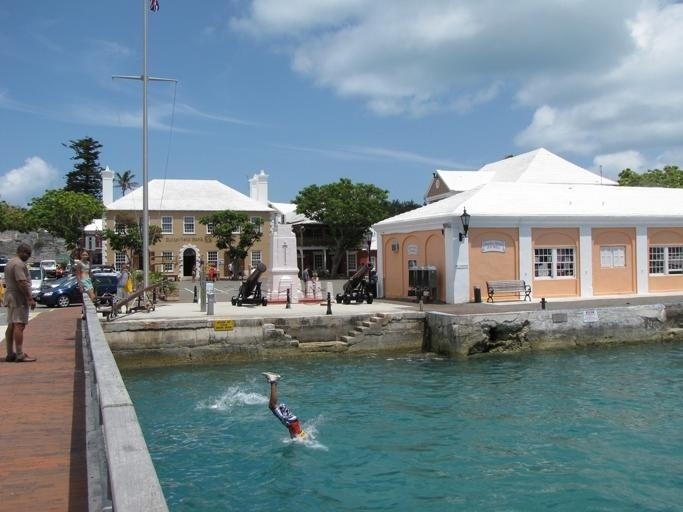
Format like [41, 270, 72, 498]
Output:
[261, 372, 280, 383]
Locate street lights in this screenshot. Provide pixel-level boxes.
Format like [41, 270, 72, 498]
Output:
[365, 228, 374, 290]
[298, 225, 305, 279]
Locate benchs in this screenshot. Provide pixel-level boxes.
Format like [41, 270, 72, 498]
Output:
[486, 280, 531, 303]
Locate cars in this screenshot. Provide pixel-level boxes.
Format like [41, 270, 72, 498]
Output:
[0, 256, 121, 310]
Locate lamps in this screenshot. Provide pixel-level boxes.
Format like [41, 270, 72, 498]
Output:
[459, 205, 471, 241]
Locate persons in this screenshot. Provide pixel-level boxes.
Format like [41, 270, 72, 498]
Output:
[76, 249, 95, 300]
[261, 371, 307, 440]
[192, 265, 197, 279]
[116, 264, 132, 298]
[228, 261, 234, 279]
[55, 266, 64, 279]
[303, 269, 309, 281]
[3, 243, 37, 362]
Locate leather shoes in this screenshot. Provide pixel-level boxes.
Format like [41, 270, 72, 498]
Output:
[15, 356, 35, 362]
[5, 353, 26, 362]
